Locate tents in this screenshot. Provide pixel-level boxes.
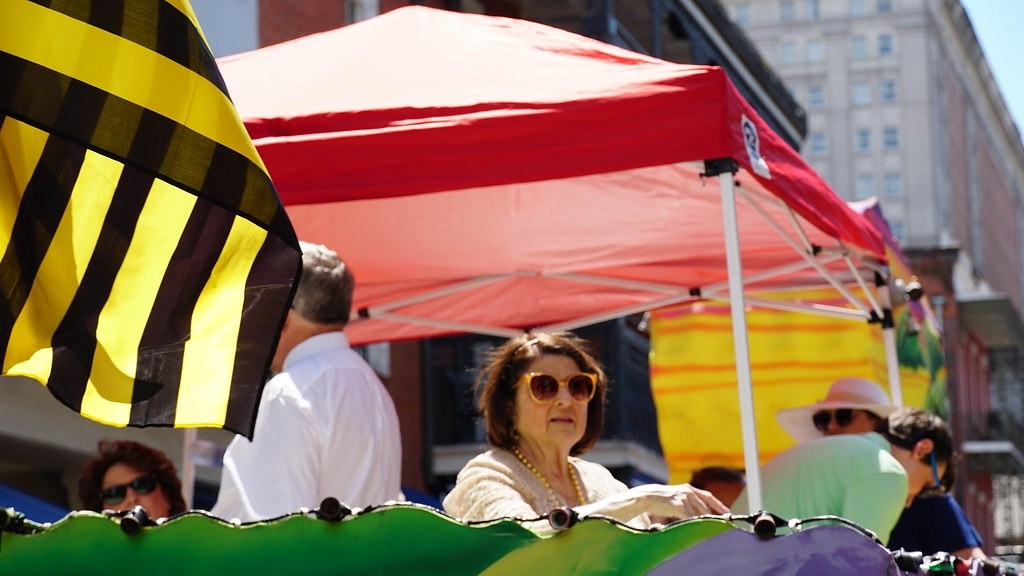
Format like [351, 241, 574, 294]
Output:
[213, 4, 945, 527]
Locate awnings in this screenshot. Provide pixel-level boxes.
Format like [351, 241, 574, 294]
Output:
[497, 0, 809, 152]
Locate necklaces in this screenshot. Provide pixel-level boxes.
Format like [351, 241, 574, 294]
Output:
[513, 448, 586, 511]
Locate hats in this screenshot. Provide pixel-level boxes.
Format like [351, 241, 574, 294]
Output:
[776, 378, 898, 444]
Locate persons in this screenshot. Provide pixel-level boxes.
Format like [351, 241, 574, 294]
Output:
[442, 329, 732, 540]
[729, 407, 956, 546]
[775, 375, 985, 560]
[690, 466, 744, 508]
[79, 438, 186, 528]
[209, 239, 402, 524]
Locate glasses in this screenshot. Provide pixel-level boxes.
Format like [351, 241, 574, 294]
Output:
[100, 473, 157, 505]
[524, 372, 598, 405]
[813, 409, 865, 431]
[914, 452, 940, 499]
